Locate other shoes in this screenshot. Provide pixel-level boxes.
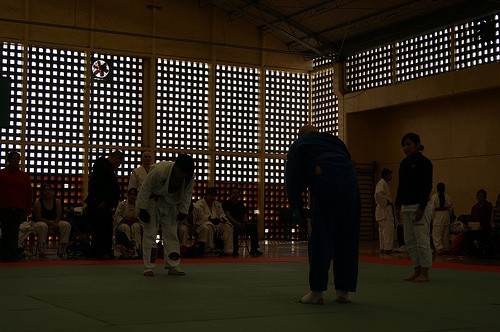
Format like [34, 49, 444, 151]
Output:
[249, 250, 263, 256]
[233, 252, 240, 257]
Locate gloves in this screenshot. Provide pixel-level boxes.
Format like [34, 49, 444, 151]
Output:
[137, 210, 151, 224]
[178, 212, 185, 221]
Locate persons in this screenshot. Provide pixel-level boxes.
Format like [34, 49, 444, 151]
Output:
[373, 168, 500, 255]
[135, 154, 195, 275]
[0, 152, 264, 262]
[286, 125, 362, 305]
[394, 133, 434, 282]
